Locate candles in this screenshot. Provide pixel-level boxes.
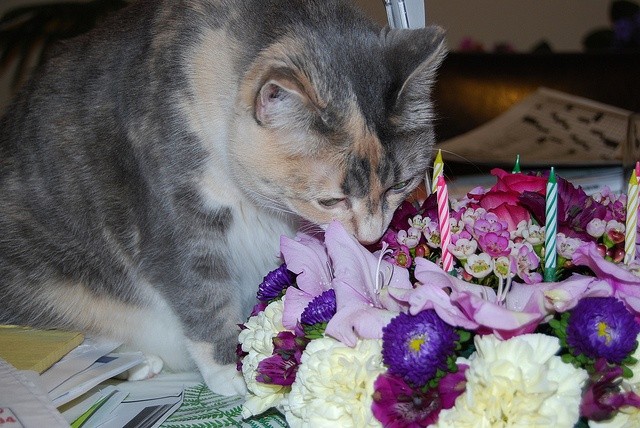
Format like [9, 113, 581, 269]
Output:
[635, 162, 640, 228]
[543, 165, 558, 283]
[511, 154, 522, 174]
[436, 175, 457, 278]
[623, 168, 638, 265]
[432, 148, 445, 193]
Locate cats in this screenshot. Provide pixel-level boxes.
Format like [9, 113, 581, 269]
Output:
[1, 1, 470, 399]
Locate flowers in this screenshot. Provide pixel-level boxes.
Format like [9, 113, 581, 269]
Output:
[236, 168, 640, 427]
